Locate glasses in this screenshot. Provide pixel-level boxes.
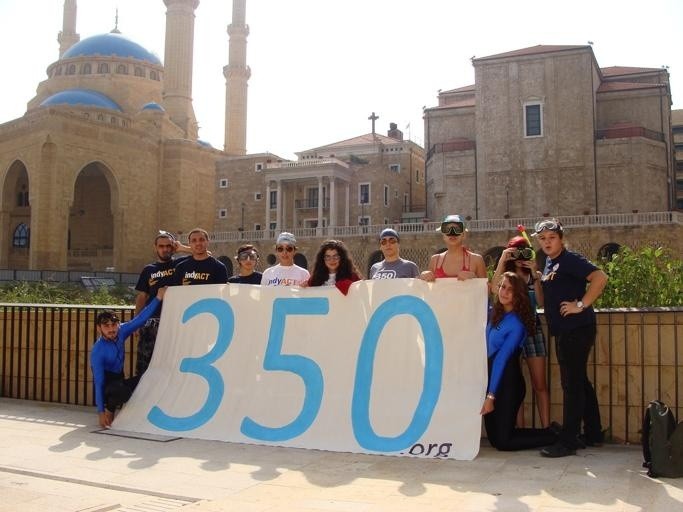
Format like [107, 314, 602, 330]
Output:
[441, 222, 465, 234]
[235, 252, 258, 261]
[381, 239, 396, 244]
[100, 315, 120, 324]
[277, 247, 294, 253]
[535, 220, 564, 233]
[511, 248, 533, 261]
[324, 254, 340, 261]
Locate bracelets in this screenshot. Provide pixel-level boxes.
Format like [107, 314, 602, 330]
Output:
[487, 394, 497, 402]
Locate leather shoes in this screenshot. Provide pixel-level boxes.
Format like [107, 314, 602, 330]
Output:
[540, 422, 602, 457]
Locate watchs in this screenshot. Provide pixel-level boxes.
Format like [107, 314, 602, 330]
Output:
[574, 297, 585, 309]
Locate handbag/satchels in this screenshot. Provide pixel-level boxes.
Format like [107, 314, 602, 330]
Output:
[637, 400, 683, 478]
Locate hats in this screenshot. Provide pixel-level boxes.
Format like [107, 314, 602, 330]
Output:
[379, 228, 398, 238]
[276, 232, 296, 246]
[508, 235, 529, 248]
[443, 214, 465, 231]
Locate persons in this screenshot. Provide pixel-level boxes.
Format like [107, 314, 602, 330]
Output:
[89, 287, 168, 429]
[172, 228, 227, 284]
[135, 232, 211, 382]
[493, 226, 552, 428]
[483, 267, 587, 452]
[533, 218, 608, 459]
[227, 210, 488, 288]
